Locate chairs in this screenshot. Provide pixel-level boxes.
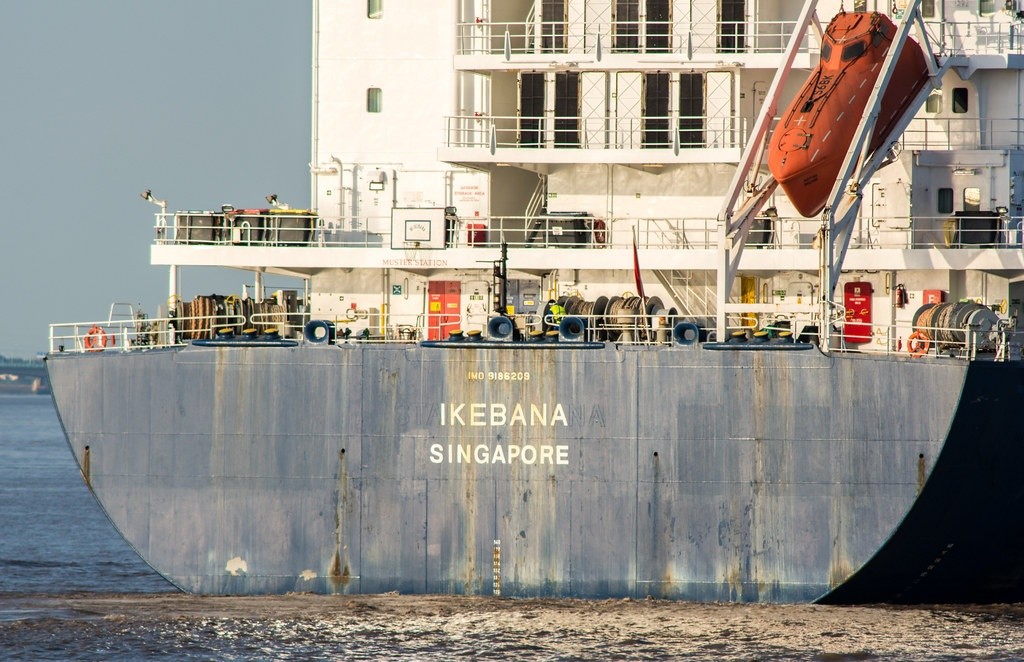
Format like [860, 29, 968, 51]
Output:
[399, 324, 415, 341]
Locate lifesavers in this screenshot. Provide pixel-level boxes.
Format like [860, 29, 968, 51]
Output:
[84, 327, 108, 353]
[907, 331, 930, 358]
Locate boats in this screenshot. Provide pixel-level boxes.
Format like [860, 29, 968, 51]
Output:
[766, 10, 931, 217]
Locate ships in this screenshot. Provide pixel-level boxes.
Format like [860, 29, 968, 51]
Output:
[44, 2, 1024, 611]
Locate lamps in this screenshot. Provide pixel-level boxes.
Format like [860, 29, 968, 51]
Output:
[221, 203, 234, 213]
[765, 206, 778, 218]
[265, 194, 289, 211]
[367, 182, 385, 191]
[140, 189, 168, 209]
[445, 206, 457, 216]
[996, 206, 1009, 215]
